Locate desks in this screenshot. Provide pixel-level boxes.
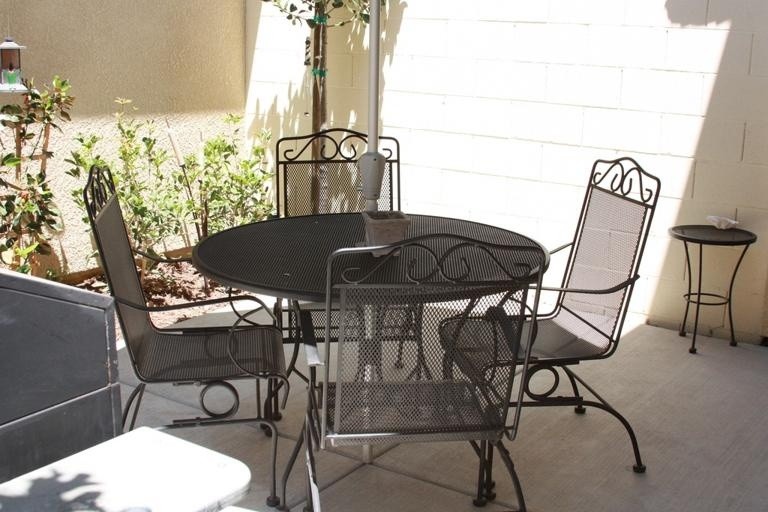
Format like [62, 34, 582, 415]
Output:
[668, 223, 758, 355]
